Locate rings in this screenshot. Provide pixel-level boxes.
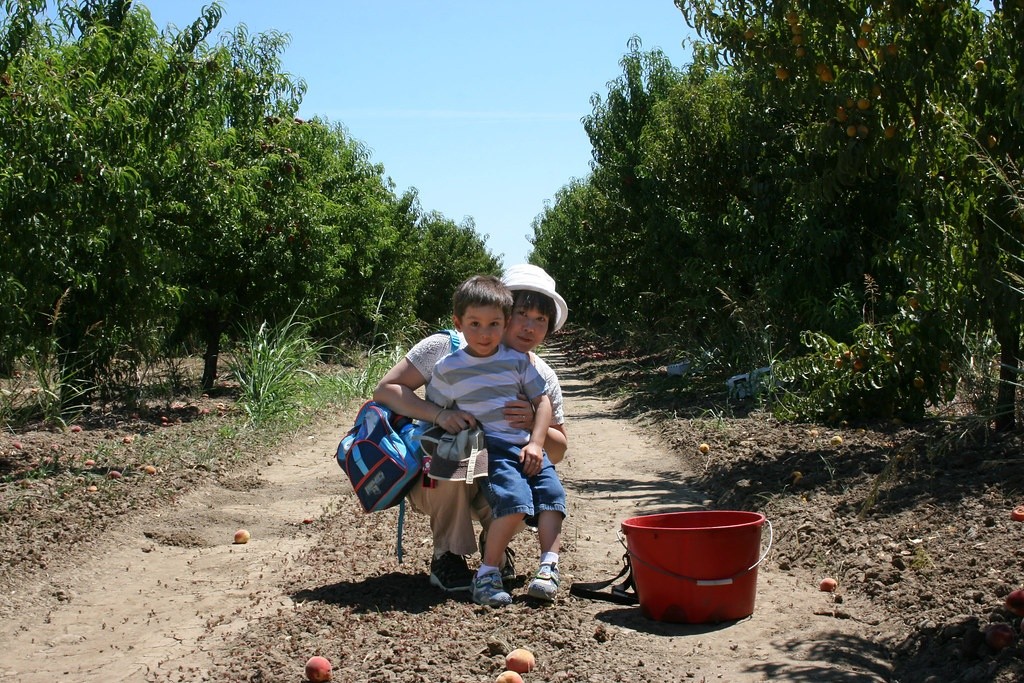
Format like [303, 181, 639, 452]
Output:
[523, 416, 526, 421]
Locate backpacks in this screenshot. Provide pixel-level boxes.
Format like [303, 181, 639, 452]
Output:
[335, 400, 423, 563]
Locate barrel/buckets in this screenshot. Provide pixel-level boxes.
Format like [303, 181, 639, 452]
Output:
[616, 510, 773, 625]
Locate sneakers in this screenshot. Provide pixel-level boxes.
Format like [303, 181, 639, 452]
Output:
[479, 530, 517, 579]
[528, 562, 559, 601]
[469, 571, 513, 607]
[430, 550, 472, 590]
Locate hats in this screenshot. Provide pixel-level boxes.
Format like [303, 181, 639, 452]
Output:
[427, 425, 490, 485]
[501, 264, 568, 335]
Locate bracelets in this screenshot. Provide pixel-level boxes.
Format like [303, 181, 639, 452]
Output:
[435, 409, 446, 425]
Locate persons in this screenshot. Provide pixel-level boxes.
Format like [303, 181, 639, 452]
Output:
[373, 265, 569, 592]
[426, 274, 566, 606]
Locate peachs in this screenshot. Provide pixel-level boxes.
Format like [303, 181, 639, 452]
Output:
[0, 13, 1024, 683]
